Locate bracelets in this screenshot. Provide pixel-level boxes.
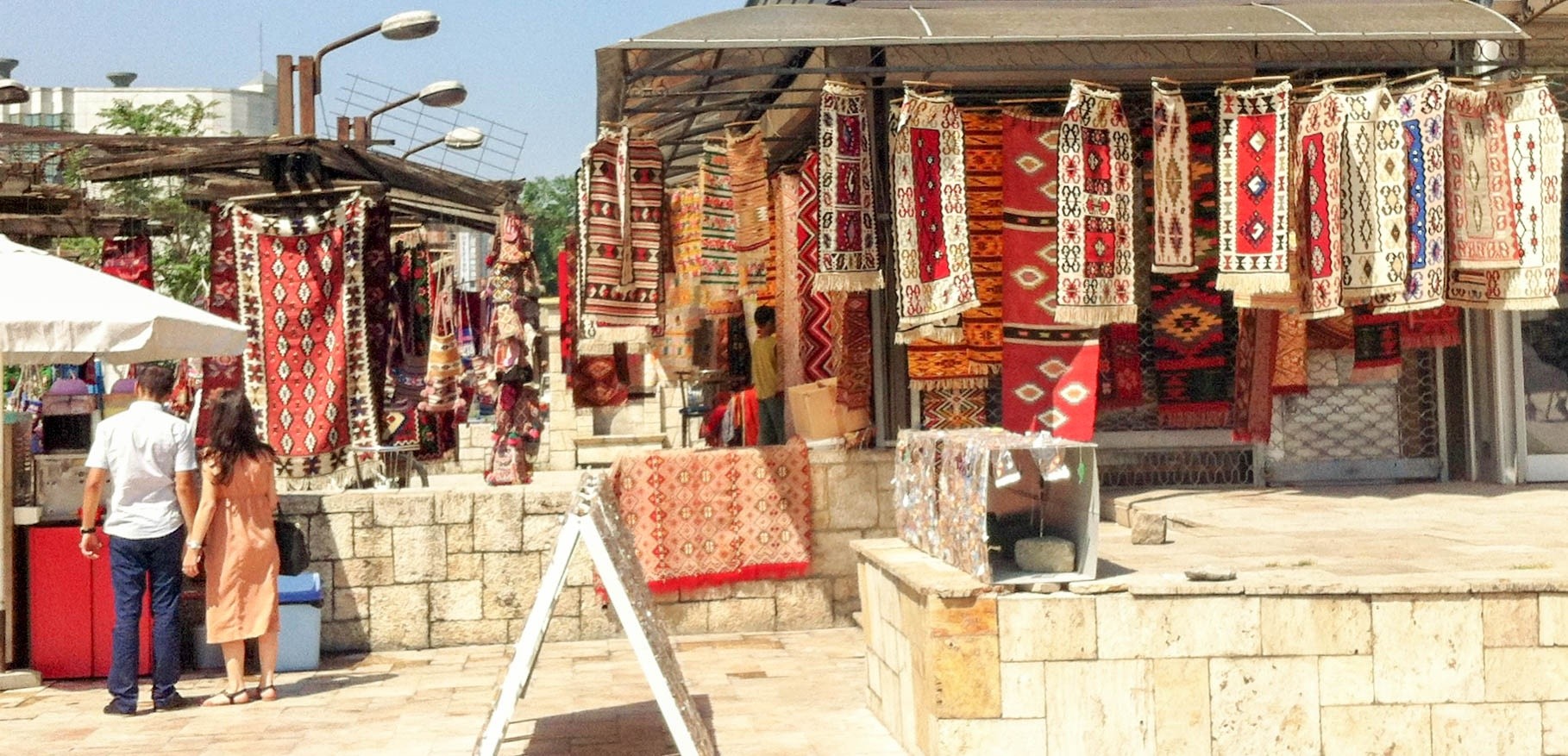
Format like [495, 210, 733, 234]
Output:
[80, 527, 96, 535]
[187, 542, 201, 551]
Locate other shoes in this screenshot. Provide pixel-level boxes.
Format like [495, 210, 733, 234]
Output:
[153, 698, 194, 712]
[103, 702, 137, 716]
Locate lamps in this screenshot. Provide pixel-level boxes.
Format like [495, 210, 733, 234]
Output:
[401, 128, 485, 160]
[314, 12, 440, 95]
[368, 80, 467, 145]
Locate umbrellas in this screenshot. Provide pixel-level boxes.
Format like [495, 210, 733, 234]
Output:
[0, 232, 248, 673]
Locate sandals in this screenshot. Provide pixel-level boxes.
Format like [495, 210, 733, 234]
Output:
[202, 688, 250, 706]
[247, 684, 277, 701]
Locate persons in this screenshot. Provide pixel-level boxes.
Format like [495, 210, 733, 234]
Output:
[79, 366, 199, 717]
[182, 389, 280, 706]
[750, 304, 786, 444]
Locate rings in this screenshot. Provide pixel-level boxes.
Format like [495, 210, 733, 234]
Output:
[181, 568, 184, 572]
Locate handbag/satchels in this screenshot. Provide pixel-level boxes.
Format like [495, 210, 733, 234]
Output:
[273, 499, 310, 577]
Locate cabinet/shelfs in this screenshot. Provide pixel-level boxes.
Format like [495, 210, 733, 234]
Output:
[27, 515, 154, 679]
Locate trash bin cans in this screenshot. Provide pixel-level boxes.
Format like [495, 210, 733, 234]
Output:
[273, 571, 323, 672]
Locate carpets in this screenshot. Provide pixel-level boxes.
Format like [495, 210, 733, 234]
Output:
[103, 79, 1563, 604]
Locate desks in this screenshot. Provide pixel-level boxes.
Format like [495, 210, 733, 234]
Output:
[891, 428, 1100, 586]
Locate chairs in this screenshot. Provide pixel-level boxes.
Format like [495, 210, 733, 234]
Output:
[345, 444, 429, 489]
[673, 369, 726, 448]
